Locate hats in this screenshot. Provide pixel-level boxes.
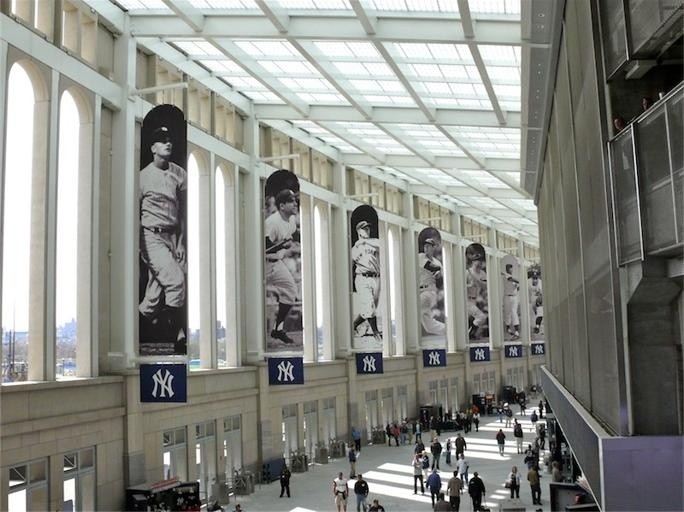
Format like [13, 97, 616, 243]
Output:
[506, 264, 512, 271]
[275, 189, 299, 205]
[471, 254, 483, 261]
[151, 125, 171, 143]
[356, 221, 370, 230]
[531, 270, 539, 278]
[424, 238, 436, 245]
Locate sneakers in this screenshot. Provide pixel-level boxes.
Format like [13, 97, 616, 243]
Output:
[354, 328, 360, 337]
[373, 331, 381, 342]
[506, 328, 514, 335]
[511, 333, 520, 341]
[470, 335, 480, 340]
[173, 336, 187, 355]
[271, 329, 296, 345]
[535, 328, 539, 334]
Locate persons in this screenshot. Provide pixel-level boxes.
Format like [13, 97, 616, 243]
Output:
[137, 120, 185, 357]
[528, 267, 544, 334]
[417, 236, 446, 336]
[500, 262, 523, 337]
[263, 187, 301, 345]
[467, 253, 487, 339]
[276, 463, 292, 497]
[330, 382, 586, 511]
[233, 504, 242, 511]
[350, 221, 381, 339]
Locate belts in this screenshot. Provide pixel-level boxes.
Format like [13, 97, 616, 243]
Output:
[469, 296, 477, 299]
[155, 227, 167, 233]
[266, 258, 279, 263]
[363, 273, 380, 277]
[420, 286, 426, 288]
